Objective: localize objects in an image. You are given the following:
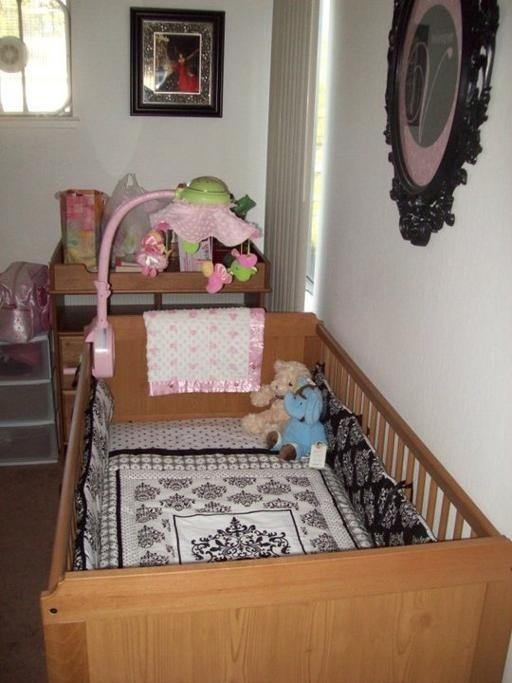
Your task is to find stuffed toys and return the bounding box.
[241,359,327,462]
[201,249,257,294]
[136,229,169,277]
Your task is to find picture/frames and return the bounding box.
[383,0,501,248]
[129,6,225,118]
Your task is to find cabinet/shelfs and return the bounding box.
[0,325,60,467]
[45,234,272,466]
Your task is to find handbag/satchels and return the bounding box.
[58,188,105,273]
[1,261,50,345]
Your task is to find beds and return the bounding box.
[37,305,512,682]
